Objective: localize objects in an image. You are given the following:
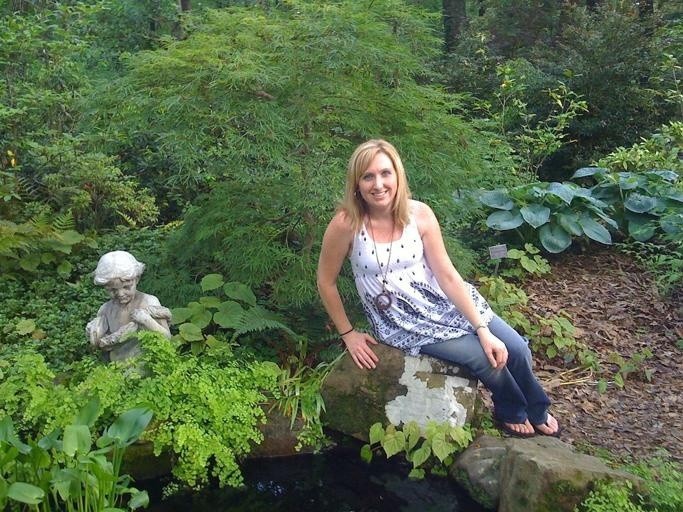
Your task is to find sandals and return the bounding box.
[501,409,536,437]
[528,410,562,437]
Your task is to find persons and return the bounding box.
[316,139,562,438]
[85,249,172,366]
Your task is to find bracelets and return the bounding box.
[339,327,354,336]
[476,323,486,332]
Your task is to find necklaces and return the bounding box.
[366,209,396,309]
